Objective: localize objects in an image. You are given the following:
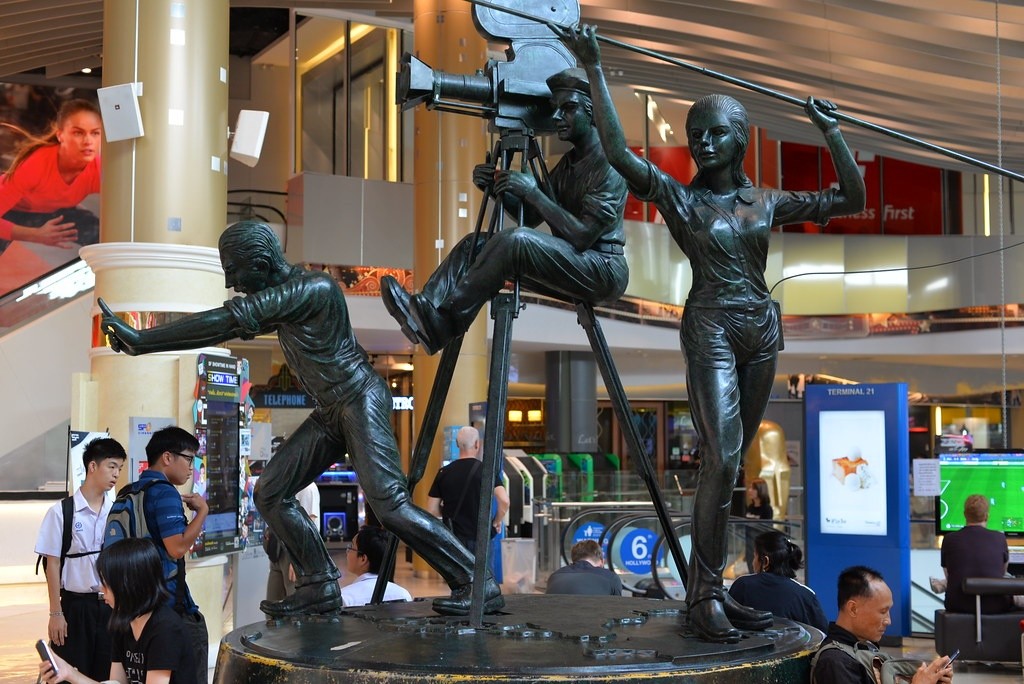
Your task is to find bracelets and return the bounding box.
[49,611,64,616]
[71,667,79,684]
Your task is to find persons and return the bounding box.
[743,477,774,573]
[729,530,832,633]
[426,427,509,578]
[927,494,1016,614]
[807,566,953,684]
[545,540,623,596]
[121,424,211,613]
[34,436,127,684]
[339,524,413,605]
[0,99,105,264]
[40,538,203,684]
[546,19,867,641]
[382,67,628,356]
[264,481,320,623]
[97,222,506,619]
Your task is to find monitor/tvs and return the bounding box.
[935,449,1024,538]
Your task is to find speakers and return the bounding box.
[229,109,271,168]
[97,84,145,143]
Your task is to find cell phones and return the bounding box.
[943,649,960,669]
[35,639,58,678]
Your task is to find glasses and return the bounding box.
[168,451,194,466]
[346,544,366,556]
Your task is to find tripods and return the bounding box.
[370,114,689,626]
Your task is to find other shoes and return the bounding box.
[929,576,946,594]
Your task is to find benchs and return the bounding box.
[935,578,1024,663]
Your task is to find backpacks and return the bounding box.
[102,479,173,552]
[810,641,925,684]
